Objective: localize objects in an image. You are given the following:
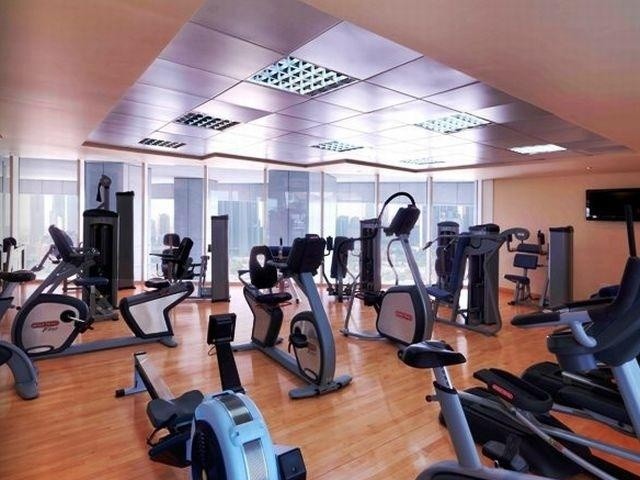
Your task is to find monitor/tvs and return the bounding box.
[586,188,640,221]
